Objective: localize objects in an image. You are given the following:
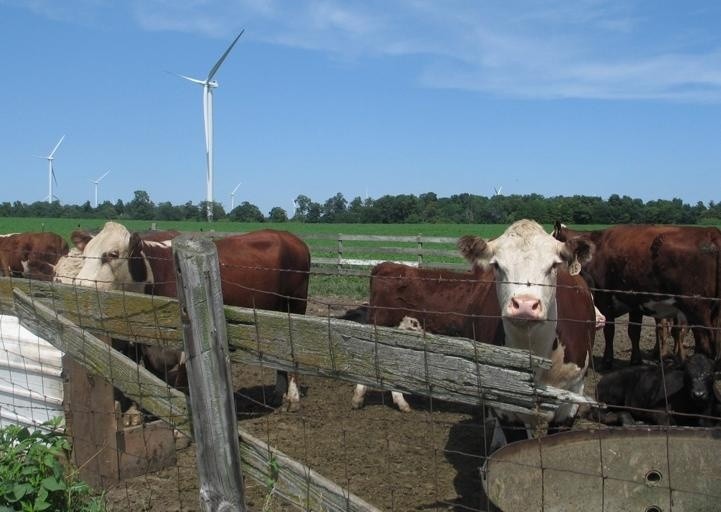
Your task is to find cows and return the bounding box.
[339,216,720,446]
[0,218,312,413]
